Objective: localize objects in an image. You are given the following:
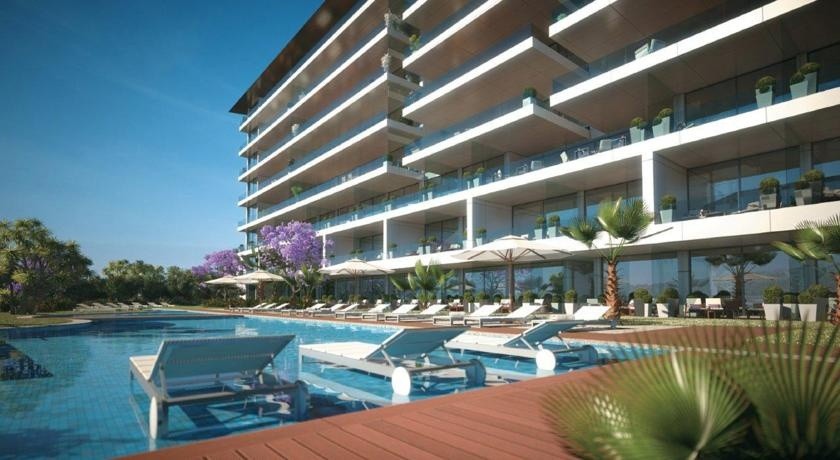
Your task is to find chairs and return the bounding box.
[76,301,176,312]
[445,320,599,370]
[229,297,764,341]
[298,326,486,396]
[129,336,308,440]
[732,201,759,214]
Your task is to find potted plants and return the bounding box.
[389,62,840,323]
